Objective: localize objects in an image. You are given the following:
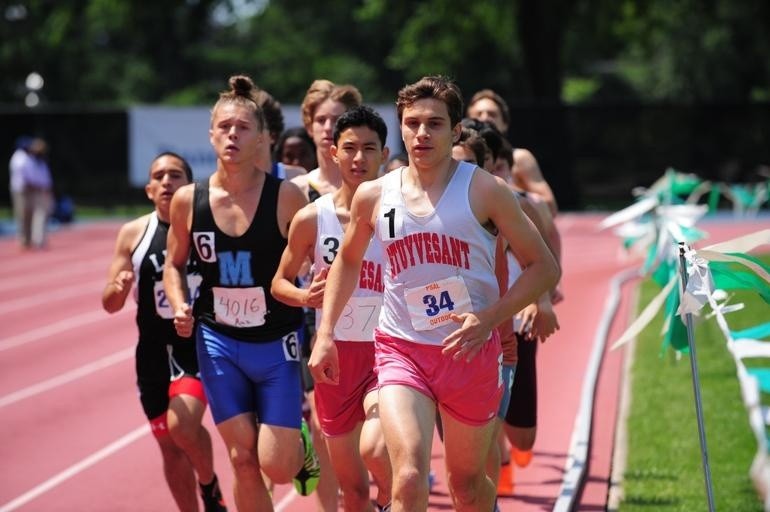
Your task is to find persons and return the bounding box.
[100,73,568,512]
[8,135,59,253]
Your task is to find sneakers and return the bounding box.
[497,463,515,496]
[511,444,533,467]
[199,474,229,512]
[294,418,321,496]
